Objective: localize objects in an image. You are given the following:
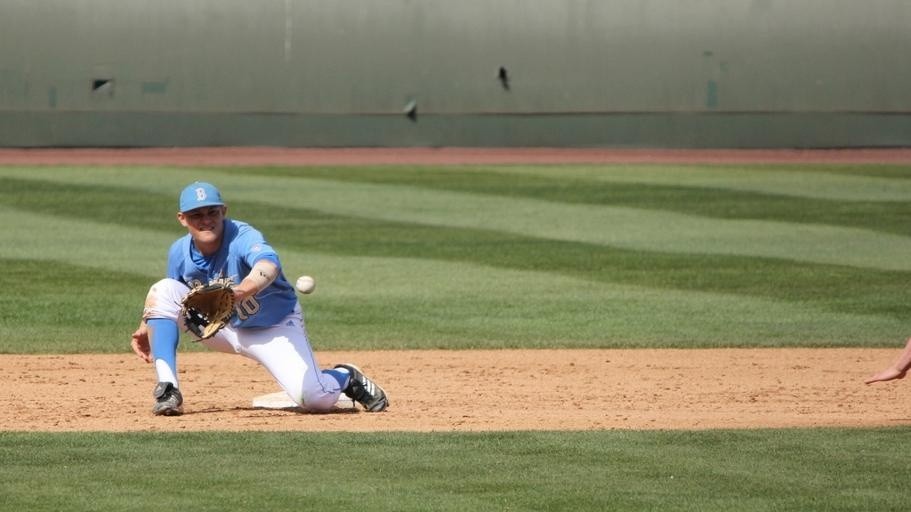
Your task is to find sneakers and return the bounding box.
[333,363,388,412]
[152,382,184,416]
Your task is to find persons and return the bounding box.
[129,179,391,416]
[863,333,911,385]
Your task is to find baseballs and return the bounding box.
[296,276,315,294]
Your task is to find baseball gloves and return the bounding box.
[174,282,234,343]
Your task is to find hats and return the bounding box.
[179,181,224,213]
